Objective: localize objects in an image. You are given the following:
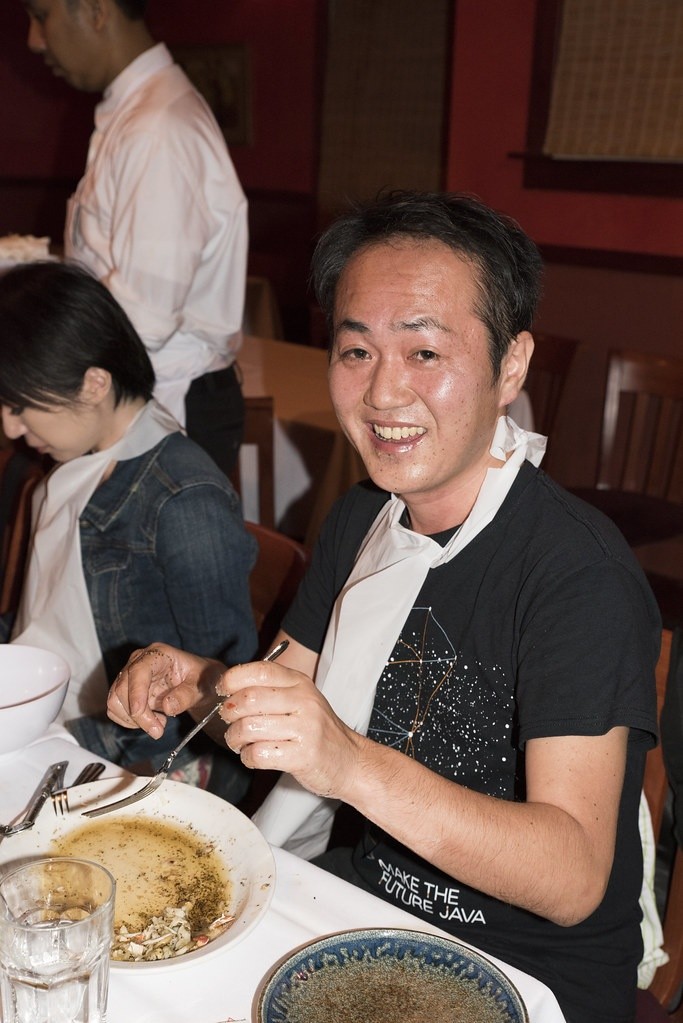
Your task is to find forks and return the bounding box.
[80,638,291,819]
[47,761,70,817]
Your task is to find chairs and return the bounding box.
[0,278,683,1023]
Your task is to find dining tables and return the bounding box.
[239,336,361,550]
[0,724,562,1023]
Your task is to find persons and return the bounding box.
[0,258,259,805]
[105,192,669,1023]
[26,0,250,479]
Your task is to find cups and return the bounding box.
[0,855,116,1023]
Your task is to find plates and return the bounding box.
[0,776,277,973]
[256,927,530,1023]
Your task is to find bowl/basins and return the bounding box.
[0,643,71,755]
